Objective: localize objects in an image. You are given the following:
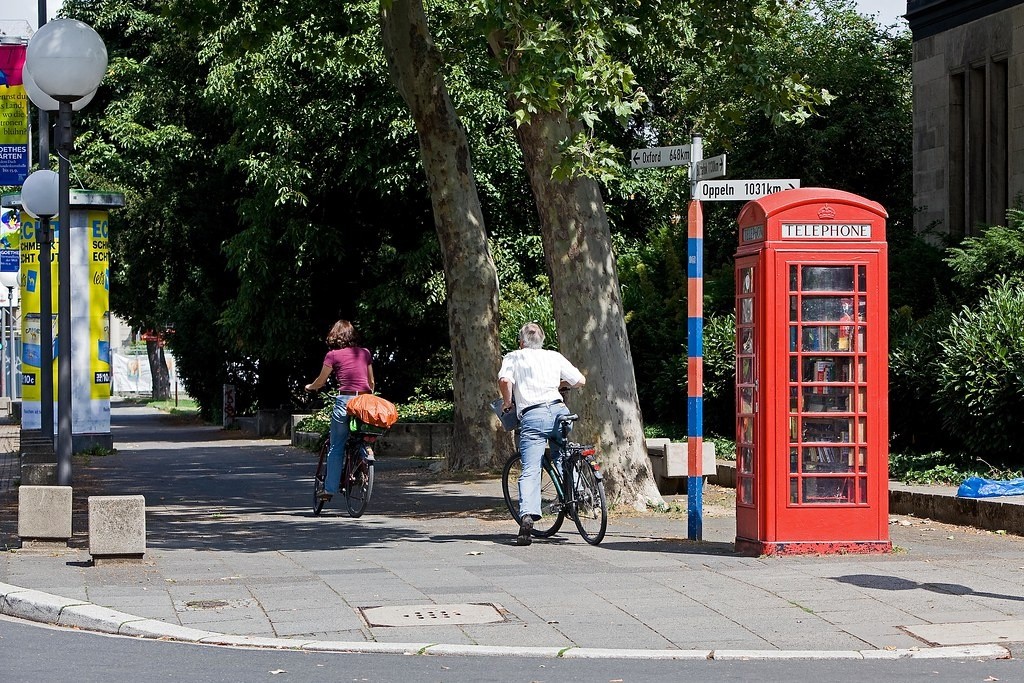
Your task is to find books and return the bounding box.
[838,297,866,351]
[813,317,839,351]
[812,359,835,394]
[807,435,836,463]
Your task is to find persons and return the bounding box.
[305,320,375,499]
[497,321,586,545]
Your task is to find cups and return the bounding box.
[812,361,831,395]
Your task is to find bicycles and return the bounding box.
[489,388,608,545]
[306,388,383,518]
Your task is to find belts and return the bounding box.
[340,390,372,395]
[521,399,563,417]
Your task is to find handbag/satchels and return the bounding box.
[346,394,399,429]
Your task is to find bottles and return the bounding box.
[837,302,851,353]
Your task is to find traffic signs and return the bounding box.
[699,179,800,201]
[631,145,690,169]
[695,154,725,179]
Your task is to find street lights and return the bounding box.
[22,19,107,484]
[21,169,59,451]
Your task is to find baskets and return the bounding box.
[491,393,519,431]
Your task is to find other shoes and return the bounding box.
[356,460,368,474]
[315,489,335,498]
[517,515,533,546]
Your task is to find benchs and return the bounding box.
[644,435,717,494]
